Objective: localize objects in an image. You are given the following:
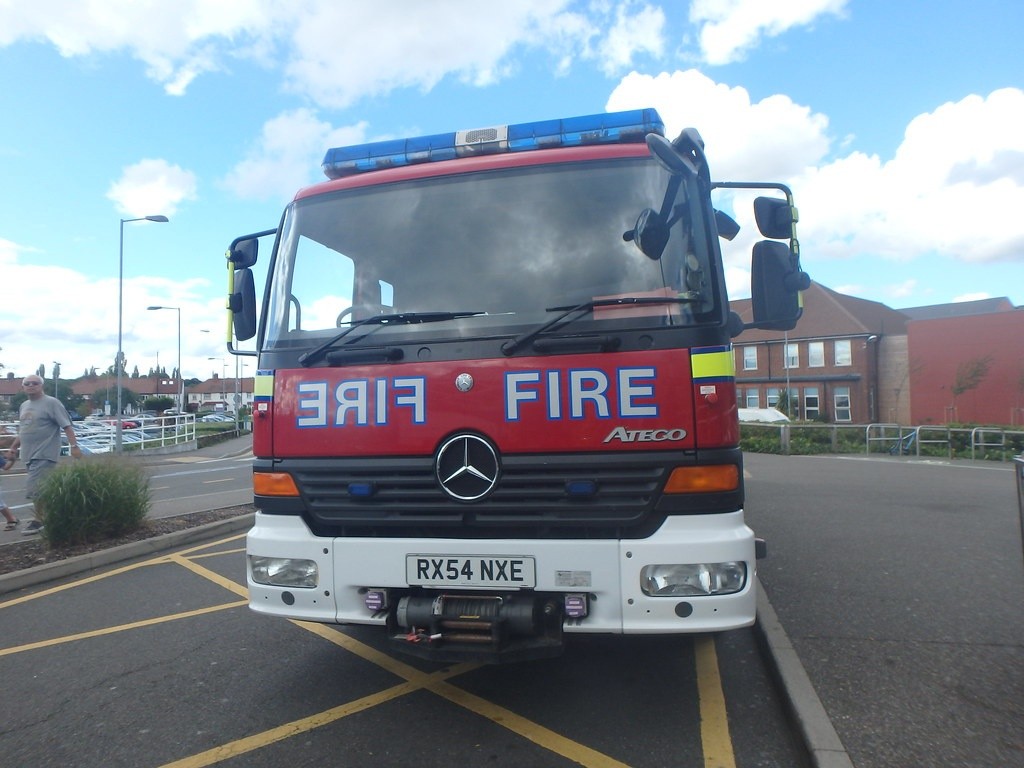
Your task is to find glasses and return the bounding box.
[23,381,43,387]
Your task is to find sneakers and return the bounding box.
[21,520,44,536]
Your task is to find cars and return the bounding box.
[0,411,237,462]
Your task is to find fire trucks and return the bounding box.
[225,107,811,669]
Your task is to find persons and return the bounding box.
[0,448,20,531]
[4,375,81,535]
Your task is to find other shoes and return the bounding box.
[5,518,19,531]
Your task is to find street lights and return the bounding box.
[53,360,61,398]
[199,328,247,435]
[147,306,181,427]
[112,215,170,455]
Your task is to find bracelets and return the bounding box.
[71,444,78,448]
